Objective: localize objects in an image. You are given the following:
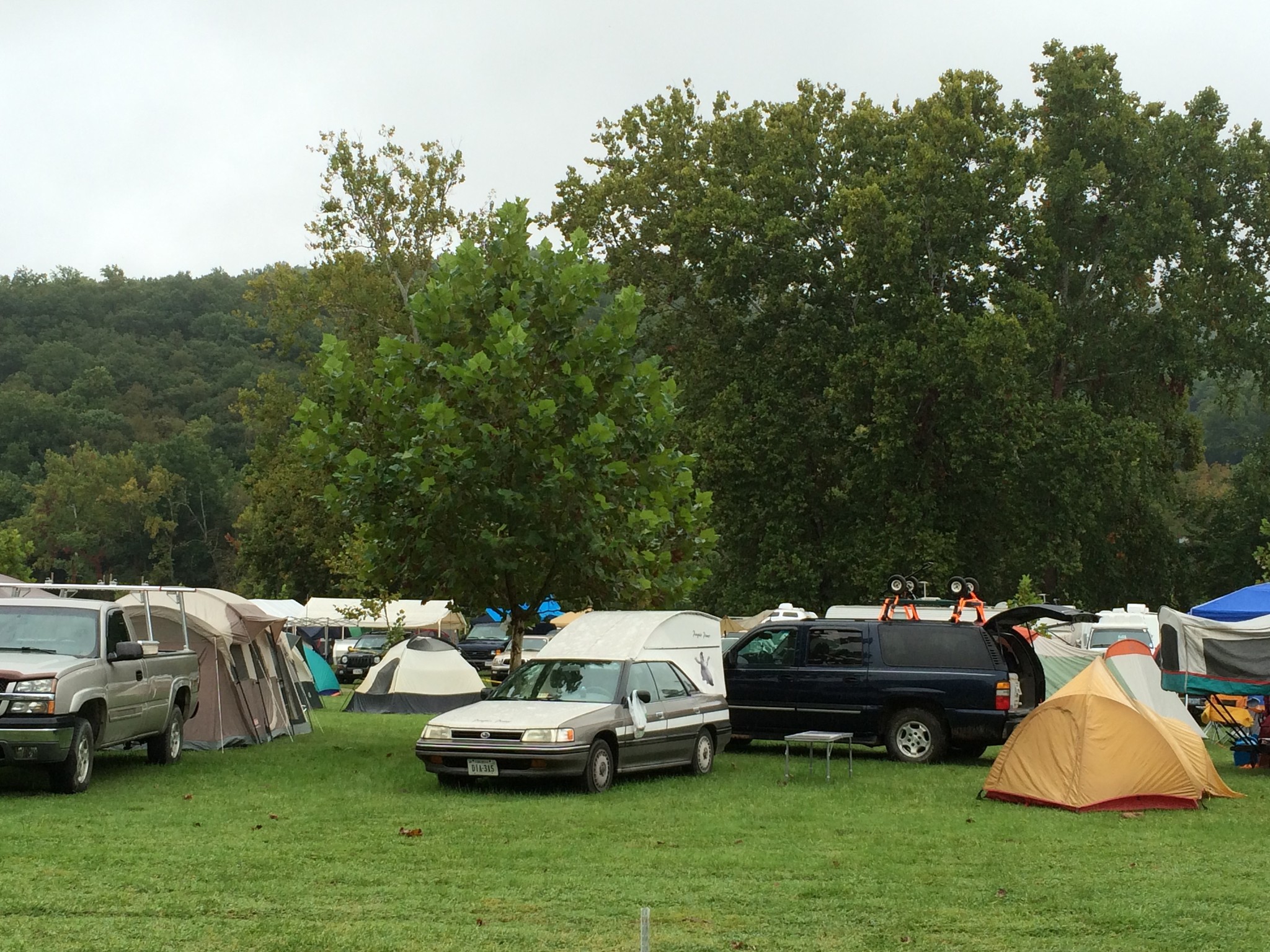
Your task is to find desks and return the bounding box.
[784,731,853,780]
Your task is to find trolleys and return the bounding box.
[878,561,987,608]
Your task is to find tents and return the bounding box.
[470,594,598,629]
[719,609,774,637]
[981,655,1250,812]
[0,573,466,754]
[343,634,490,713]
[1007,578,1270,750]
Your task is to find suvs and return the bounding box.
[334,630,414,684]
[720,604,1102,765]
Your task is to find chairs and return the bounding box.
[1203,696,1264,746]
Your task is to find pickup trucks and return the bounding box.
[0,579,203,796]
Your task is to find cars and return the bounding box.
[413,654,733,795]
[1078,624,1156,657]
[456,621,513,669]
[488,635,553,687]
